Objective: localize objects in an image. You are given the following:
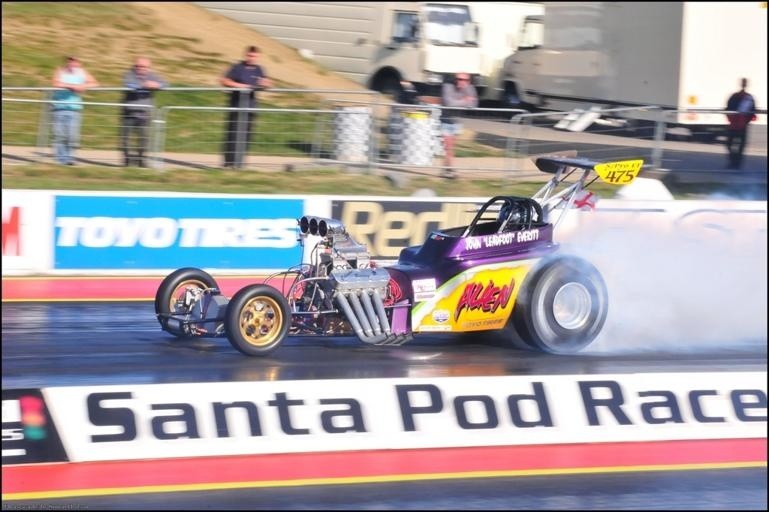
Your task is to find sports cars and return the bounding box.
[154,153,645,358]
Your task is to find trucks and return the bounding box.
[502,2,768,137]
[188,3,487,103]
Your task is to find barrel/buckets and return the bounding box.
[330,104,375,173]
[386,106,444,169]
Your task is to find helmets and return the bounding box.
[497,198,543,232]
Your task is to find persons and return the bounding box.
[219,43,274,169]
[434,70,481,180]
[47,53,100,169]
[117,57,170,170]
[721,77,757,171]
[492,204,532,233]
